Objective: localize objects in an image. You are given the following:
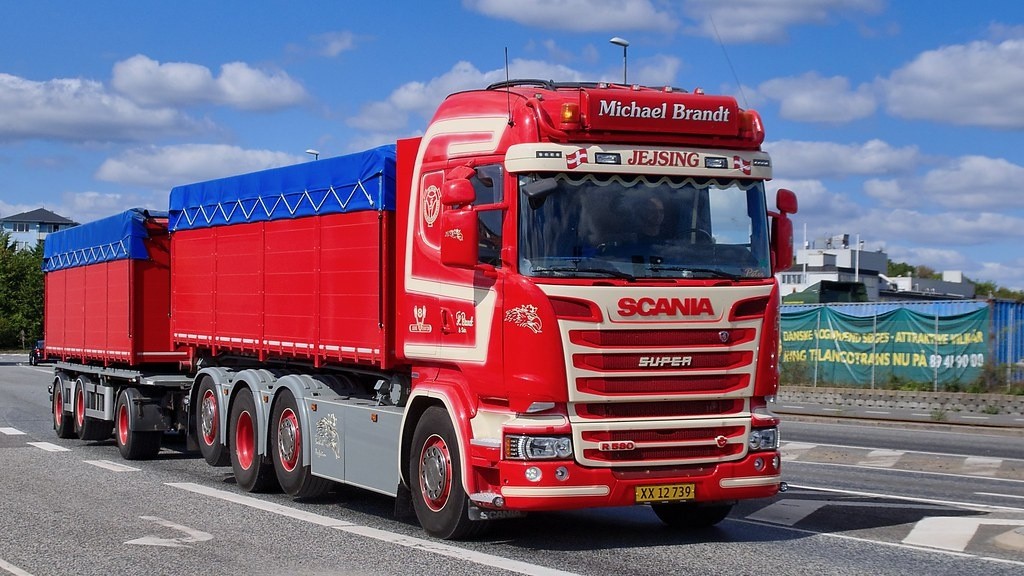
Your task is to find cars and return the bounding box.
[29,339,62,366]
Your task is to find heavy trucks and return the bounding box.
[37,80,801,543]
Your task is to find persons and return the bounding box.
[640,197,665,246]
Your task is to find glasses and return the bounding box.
[647,207,666,214]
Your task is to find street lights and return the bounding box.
[609,36,630,85]
